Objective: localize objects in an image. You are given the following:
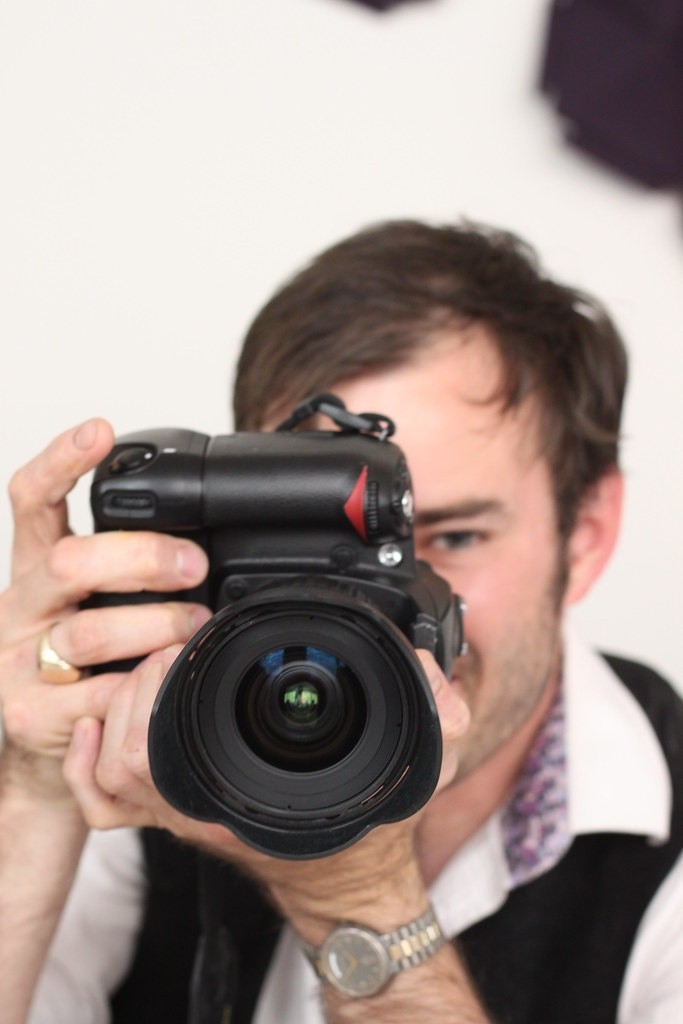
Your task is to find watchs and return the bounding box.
[303,904,446,1003]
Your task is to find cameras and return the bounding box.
[76,424,464,860]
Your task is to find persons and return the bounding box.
[0,209,683,1024]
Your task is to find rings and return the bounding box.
[37,619,81,687]
